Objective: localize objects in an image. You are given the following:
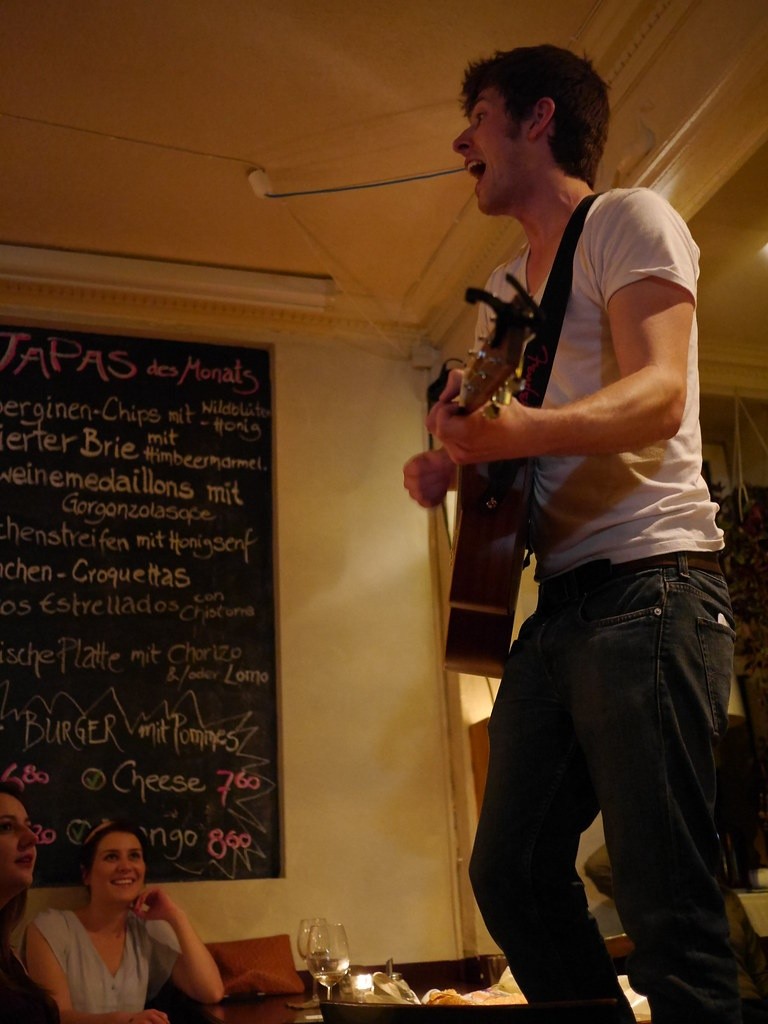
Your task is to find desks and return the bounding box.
[195,974,489,1024]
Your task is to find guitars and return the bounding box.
[441,272,543,679]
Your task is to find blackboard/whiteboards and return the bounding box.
[0,313,289,884]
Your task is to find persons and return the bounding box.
[1,780,223,1024]
[403,45,768,1024]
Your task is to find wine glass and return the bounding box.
[307,923,350,1001]
[298,917,330,1003]
